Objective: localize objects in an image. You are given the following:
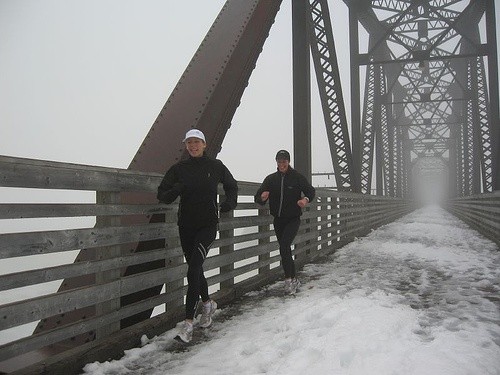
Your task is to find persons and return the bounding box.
[156,129,239,343]
[254,150,316,297]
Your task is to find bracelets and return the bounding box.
[304,197,309,203]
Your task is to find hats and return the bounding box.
[276,150,290,161]
[182,129,206,143]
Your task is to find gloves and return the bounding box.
[173,182,184,193]
[219,201,235,213]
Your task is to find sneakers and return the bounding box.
[284,277,300,295]
[199,299,217,327]
[173,322,193,342]
